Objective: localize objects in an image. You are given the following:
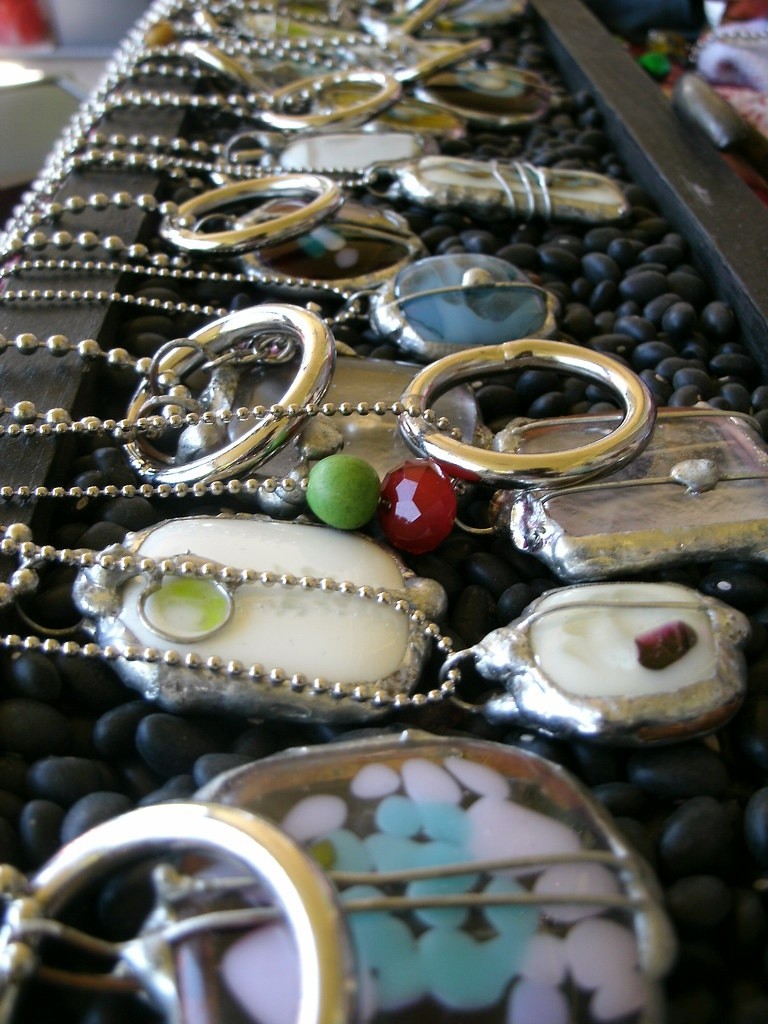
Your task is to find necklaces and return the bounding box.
[0,1,767,1024]
